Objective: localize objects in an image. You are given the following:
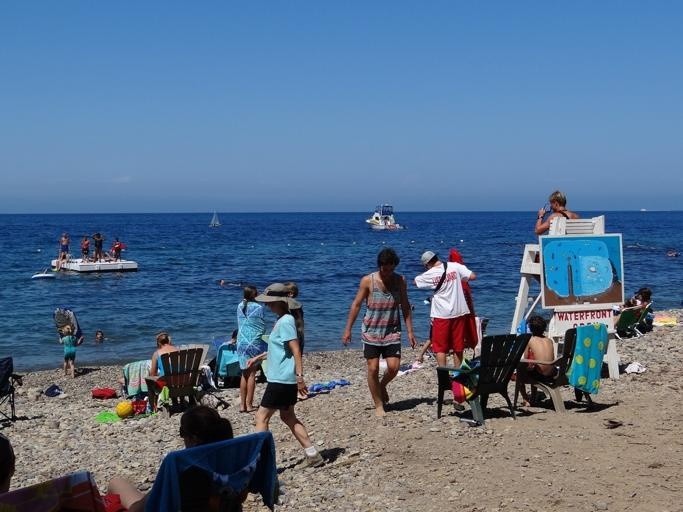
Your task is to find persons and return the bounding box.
[236,287,260,413]
[58,325,81,374]
[412,250,475,370]
[342,249,417,418]
[418,316,432,365]
[95,330,105,343]
[613,286,653,330]
[216,329,237,361]
[534,190,577,262]
[149,331,187,411]
[59,233,125,262]
[247,284,322,468]
[516,315,556,406]
[282,284,306,400]
[0,433,14,493]
[107,405,232,511]
[219,279,225,286]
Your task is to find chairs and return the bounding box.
[145,348,204,418]
[520,213,606,275]
[618,301,654,338]
[145,432,280,511]
[513,324,607,413]
[436,334,532,424]
[0,356,24,423]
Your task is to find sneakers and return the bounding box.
[294,451,323,470]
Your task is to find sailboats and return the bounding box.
[209,209,223,229]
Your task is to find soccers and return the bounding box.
[116,401,134,418]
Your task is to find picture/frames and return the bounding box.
[539,232,624,310]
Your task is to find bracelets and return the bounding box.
[537,216,543,219]
[295,373,304,377]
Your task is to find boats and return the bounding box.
[365,203,405,232]
[51,256,138,273]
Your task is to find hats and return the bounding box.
[421,251,438,266]
[635,288,651,294]
[255,283,302,310]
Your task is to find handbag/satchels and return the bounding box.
[92,388,116,400]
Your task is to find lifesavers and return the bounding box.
[32,273,56,279]
